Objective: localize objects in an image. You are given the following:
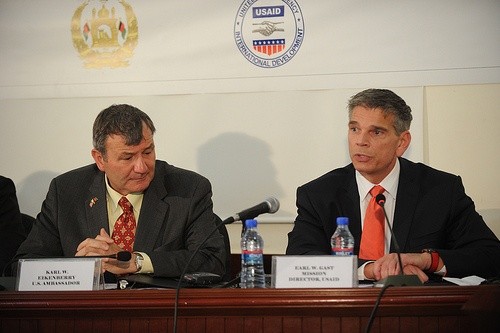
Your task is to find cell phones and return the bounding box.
[184,273,222,284]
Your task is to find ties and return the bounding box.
[110,197,136,253]
[359,184,386,261]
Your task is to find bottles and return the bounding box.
[240,220,265,289]
[330,217,355,255]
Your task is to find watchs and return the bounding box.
[135,253,145,271]
[421,248,439,272]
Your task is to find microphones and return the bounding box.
[374,194,424,287]
[224,197,280,224]
[0,250,132,291]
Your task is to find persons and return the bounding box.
[285,88,500,283]
[0,175,28,277]
[7,104,231,286]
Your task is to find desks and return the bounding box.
[0,279,500,333]
